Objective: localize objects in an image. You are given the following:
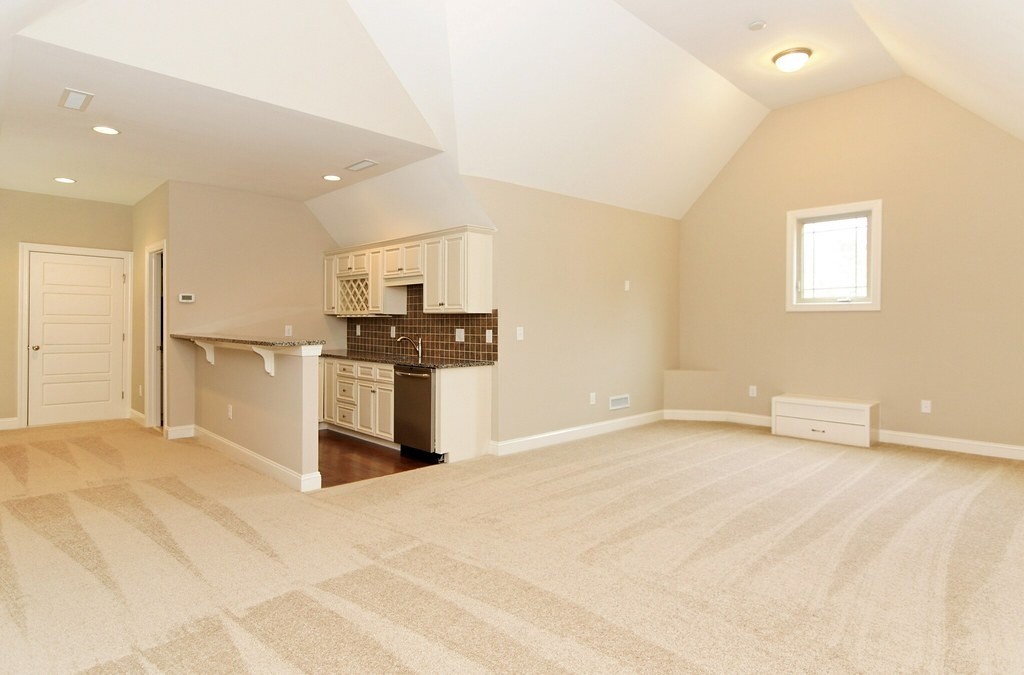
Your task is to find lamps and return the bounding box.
[772,47,813,72]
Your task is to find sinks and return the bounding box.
[380,355,426,364]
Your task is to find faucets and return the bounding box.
[397,336,421,363]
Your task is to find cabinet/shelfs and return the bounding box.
[771,393,880,449]
[319,348,495,463]
[322,224,498,316]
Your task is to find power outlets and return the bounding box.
[356,325,360,335]
[749,385,757,397]
[485,329,493,343]
[590,392,596,404]
[285,325,292,336]
[517,327,524,341]
[391,326,396,338]
[921,400,932,413]
[455,329,464,341]
